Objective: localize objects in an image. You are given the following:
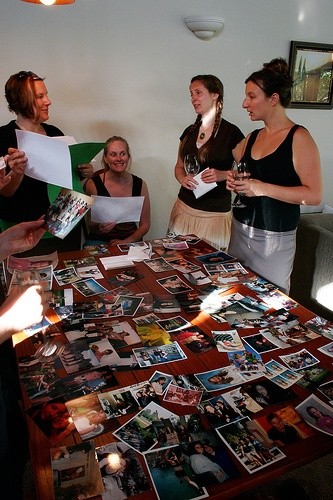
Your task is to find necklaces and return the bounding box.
[199,121,215,139]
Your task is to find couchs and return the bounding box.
[287,212,332,323]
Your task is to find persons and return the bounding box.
[165,74,247,252]
[0,70,94,258]
[0,215,51,345]
[7,186,333,500]
[83,136,150,246]
[225,57,322,298]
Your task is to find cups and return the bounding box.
[183,153,200,179]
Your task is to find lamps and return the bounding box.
[184,15,225,41]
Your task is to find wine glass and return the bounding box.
[231,160,249,207]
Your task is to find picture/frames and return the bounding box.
[284,40,333,109]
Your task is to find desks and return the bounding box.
[6,232,333,500]
